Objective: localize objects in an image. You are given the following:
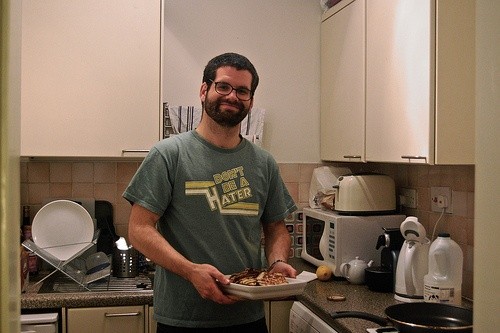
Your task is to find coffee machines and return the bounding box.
[367,226,405,291]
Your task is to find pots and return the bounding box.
[330,302,473,333]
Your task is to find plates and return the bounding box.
[216,275,306,299]
[32,200,94,261]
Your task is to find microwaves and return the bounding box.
[301,208,406,276]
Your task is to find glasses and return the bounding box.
[209,79,254,101]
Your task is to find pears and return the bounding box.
[316,265,331,281]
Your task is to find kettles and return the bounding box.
[394,216,431,303]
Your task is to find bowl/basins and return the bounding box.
[86,252,110,274]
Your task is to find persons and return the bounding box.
[122,53,297,332]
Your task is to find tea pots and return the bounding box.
[340,256,374,284]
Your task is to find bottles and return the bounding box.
[22,206,37,281]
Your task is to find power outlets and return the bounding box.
[430,186,453,214]
[399,189,417,209]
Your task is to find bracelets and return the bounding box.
[268,260,286,271]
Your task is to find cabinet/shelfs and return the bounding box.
[319,0,476,165]
[21,0,164,162]
[21,258,474,333]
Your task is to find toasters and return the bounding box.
[333,173,396,215]
